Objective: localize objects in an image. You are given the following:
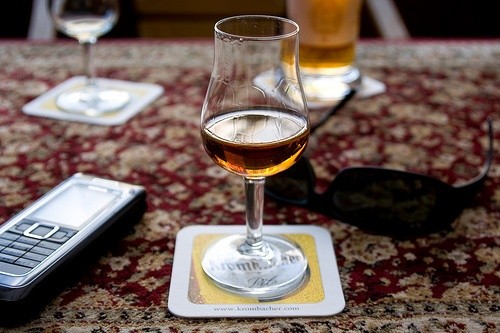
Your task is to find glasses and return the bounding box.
[264,87,493,240]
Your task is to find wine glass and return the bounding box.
[200,16,309,299]
[52,0,132,115]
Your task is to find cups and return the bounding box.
[278,0,361,87]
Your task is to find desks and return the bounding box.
[0,38,500,333]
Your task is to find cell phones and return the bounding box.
[0,172,147,302]
